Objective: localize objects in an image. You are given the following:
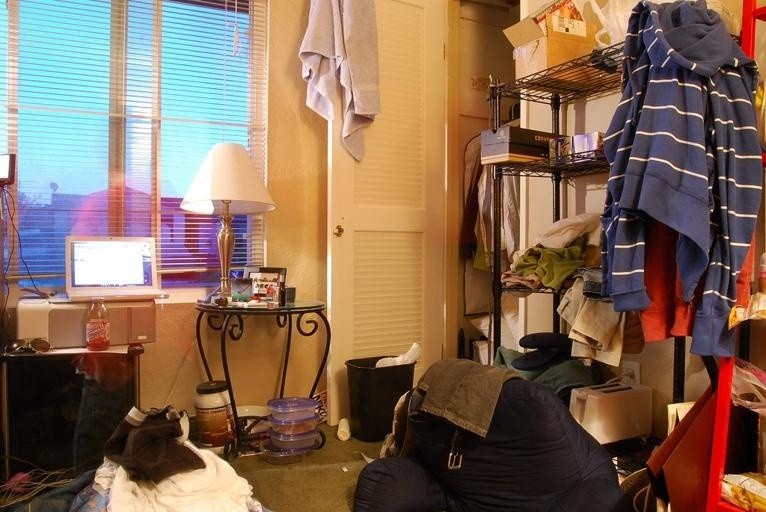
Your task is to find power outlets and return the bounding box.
[621,361,641,385]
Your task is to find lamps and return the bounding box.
[178,142,277,298]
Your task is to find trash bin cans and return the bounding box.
[344,355,417,442]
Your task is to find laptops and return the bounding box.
[64,234,170,301]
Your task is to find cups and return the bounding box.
[285,285,296,304]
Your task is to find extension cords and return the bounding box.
[47,293,68,303]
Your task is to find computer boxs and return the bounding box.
[15,298,157,350]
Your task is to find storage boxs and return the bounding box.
[502,11,598,96]
[479,126,606,165]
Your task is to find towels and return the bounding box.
[298,0,380,164]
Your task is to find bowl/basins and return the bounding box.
[230,405,272,436]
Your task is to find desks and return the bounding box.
[1,345,145,480]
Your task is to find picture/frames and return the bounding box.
[242,266,287,302]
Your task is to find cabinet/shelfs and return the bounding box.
[487,38,719,402]
[707,356,766,512]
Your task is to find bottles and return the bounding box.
[84,295,112,351]
[192,381,240,456]
[277,272,287,307]
